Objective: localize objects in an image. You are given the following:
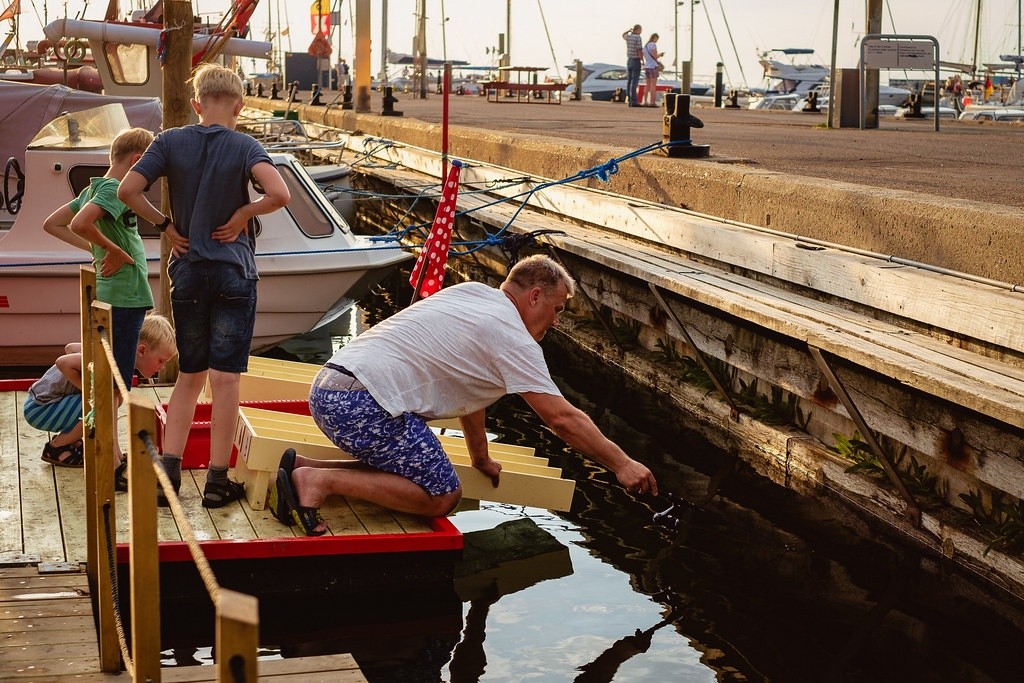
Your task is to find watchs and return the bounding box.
[153,215,171,232]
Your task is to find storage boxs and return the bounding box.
[638,84,673,104]
[154,399,309,469]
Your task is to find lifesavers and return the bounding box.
[64,39,87,62]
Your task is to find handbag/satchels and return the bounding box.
[656,61,665,72]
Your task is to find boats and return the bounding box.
[0,0,415,378]
[566,45,1023,123]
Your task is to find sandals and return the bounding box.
[114,462,128,494]
[157,479,181,507]
[202,477,245,508]
[52,434,83,455]
[41,441,85,468]
[120,452,128,464]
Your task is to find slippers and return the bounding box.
[277,466,327,536]
[268,448,296,524]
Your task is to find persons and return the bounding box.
[116,64,290,507]
[644,33,663,107]
[339,59,348,91]
[622,24,644,107]
[24,315,178,467]
[269,253,657,537]
[43,128,156,490]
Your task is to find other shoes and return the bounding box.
[642,103,648,106]
[629,103,643,107]
[648,103,659,108]
